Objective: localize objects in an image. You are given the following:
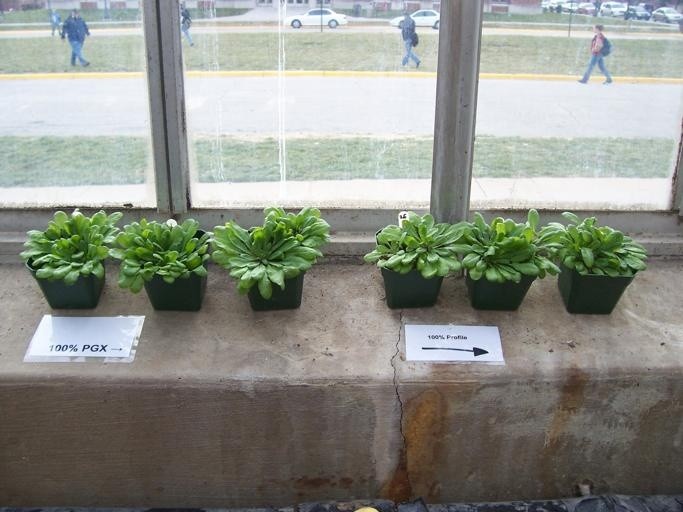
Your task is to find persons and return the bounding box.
[578,24,613,83]
[61,10,90,68]
[180,3,196,46]
[49,9,61,37]
[399,10,421,68]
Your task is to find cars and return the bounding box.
[651,7,682,22]
[540,1,653,21]
[389,9,440,30]
[283,8,347,28]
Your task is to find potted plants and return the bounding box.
[20,206,121,308]
[550,210,648,317]
[461,210,560,310]
[205,204,334,313]
[364,209,473,309]
[111,215,212,309]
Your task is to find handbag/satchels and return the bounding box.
[410,32,419,47]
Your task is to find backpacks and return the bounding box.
[601,36,613,57]
